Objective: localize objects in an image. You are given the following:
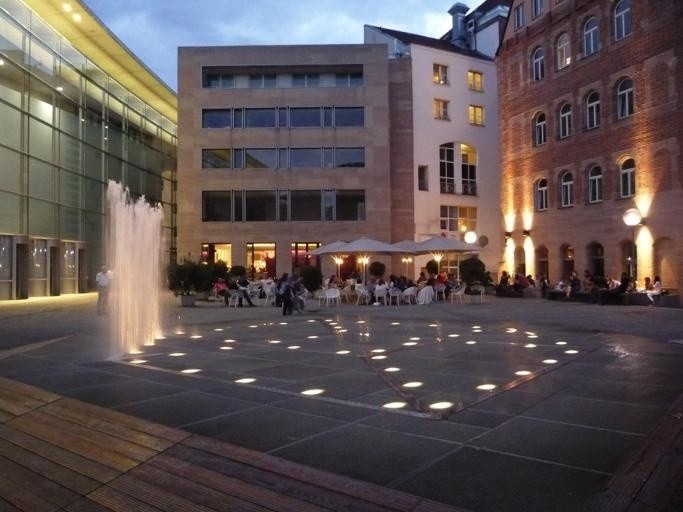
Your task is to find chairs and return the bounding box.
[211,275,486,310]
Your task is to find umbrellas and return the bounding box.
[303,237,486,289]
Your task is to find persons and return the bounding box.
[95,266,112,316]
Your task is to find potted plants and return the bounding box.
[163,250,227,307]
